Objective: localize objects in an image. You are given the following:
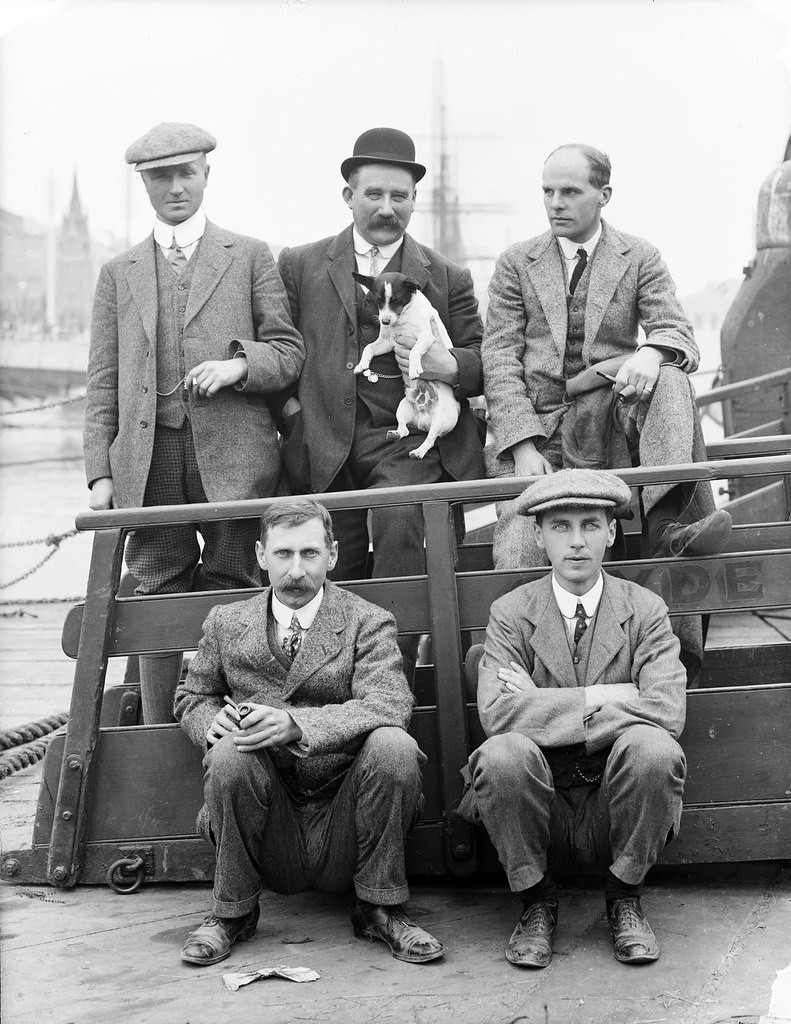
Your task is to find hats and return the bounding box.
[126,122,216,171]
[341,128,426,182]
[514,467,633,517]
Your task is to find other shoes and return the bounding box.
[464,644,484,703]
[649,509,732,560]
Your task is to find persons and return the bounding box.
[466,141,732,702]
[278,128,484,703]
[181,498,445,965]
[456,467,687,969]
[83,122,307,724]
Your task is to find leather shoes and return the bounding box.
[350,900,446,963]
[505,900,556,966]
[607,896,660,961]
[180,902,261,965]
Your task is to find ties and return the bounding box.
[281,616,302,660]
[574,603,588,645]
[167,237,187,273]
[369,248,379,277]
[569,249,587,296]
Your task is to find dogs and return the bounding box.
[352,268,462,459]
